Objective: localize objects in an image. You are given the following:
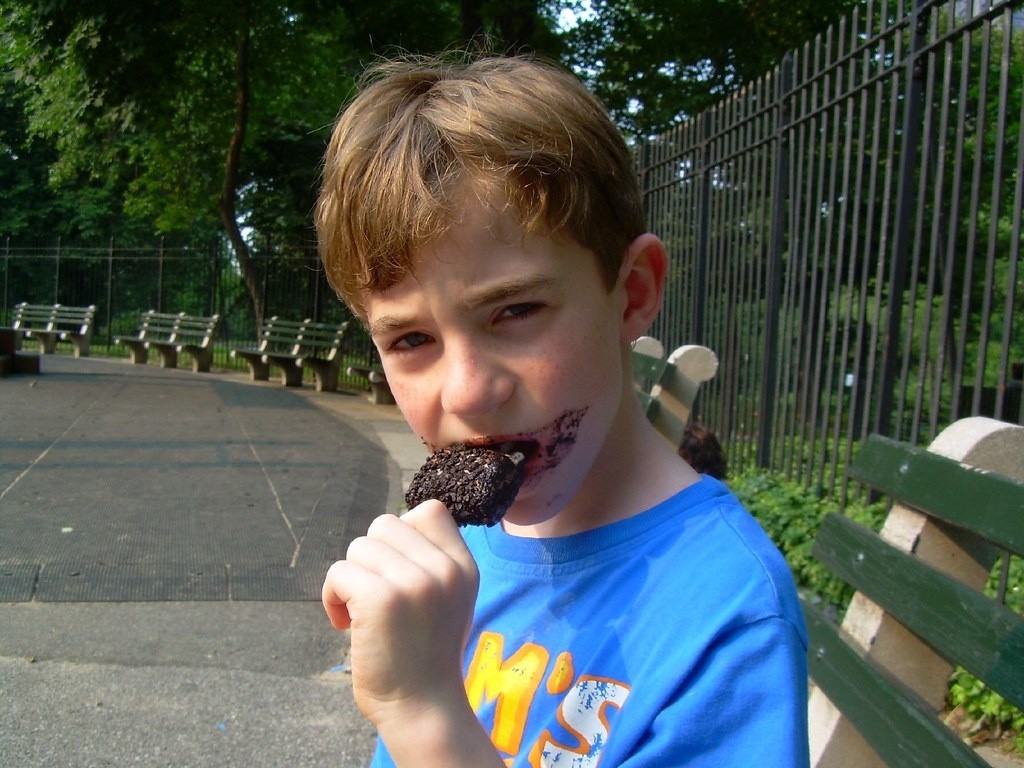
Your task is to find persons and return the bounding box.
[318,48,811,768]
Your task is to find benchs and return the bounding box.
[113,309,222,373]
[0,302,96,358]
[794,414,1024,768]
[231,316,352,391]
[631,335,718,452]
[345,338,395,404]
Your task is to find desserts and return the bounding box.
[400,440,529,521]
